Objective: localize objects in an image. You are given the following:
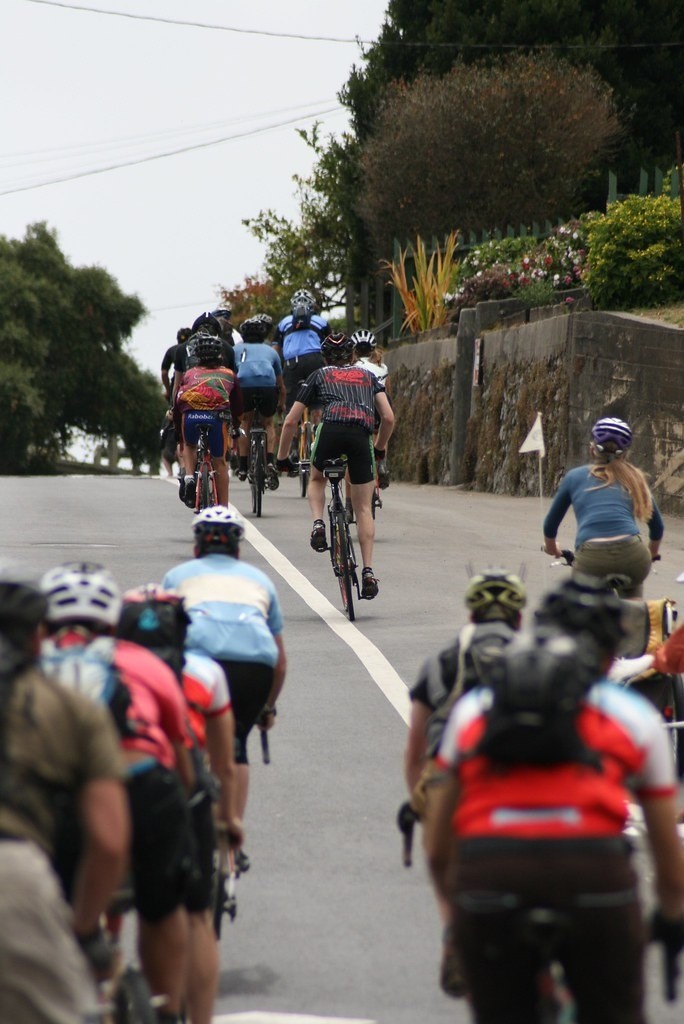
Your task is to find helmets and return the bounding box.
[193,505,243,541]
[0,560,47,623]
[253,313,271,326]
[176,327,193,344]
[193,313,221,331]
[238,318,267,335]
[319,334,354,360]
[350,328,377,350]
[195,336,222,357]
[116,584,186,636]
[212,308,231,321]
[42,561,120,624]
[290,290,316,313]
[593,416,633,447]
[465,571,525,616]
[539,584,622,658]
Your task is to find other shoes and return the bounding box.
[310,525,326,550]
[362,574,378,596]
[232,850,250,873]
[178,476,186,499]
[185,479,196,507]
[377,460,390,490]
[266,463,280,489]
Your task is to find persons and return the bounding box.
[272,288,333,476]
[160,504,286,875]
[403,567,684,1023]
[162,301,286,508]
[0,563,245,1024]
[351,328,391,489]
[542,417,663,599]
[276,331,395,596]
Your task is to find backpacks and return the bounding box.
[452,634,605,777]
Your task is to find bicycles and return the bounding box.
[213,706,268,937]
[246,396,274,517]
[194,421,218,514]
[96,883,166,1024]
[396,793,424,870]
[289,401,384,622]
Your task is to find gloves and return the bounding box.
[277,459,293,472]
